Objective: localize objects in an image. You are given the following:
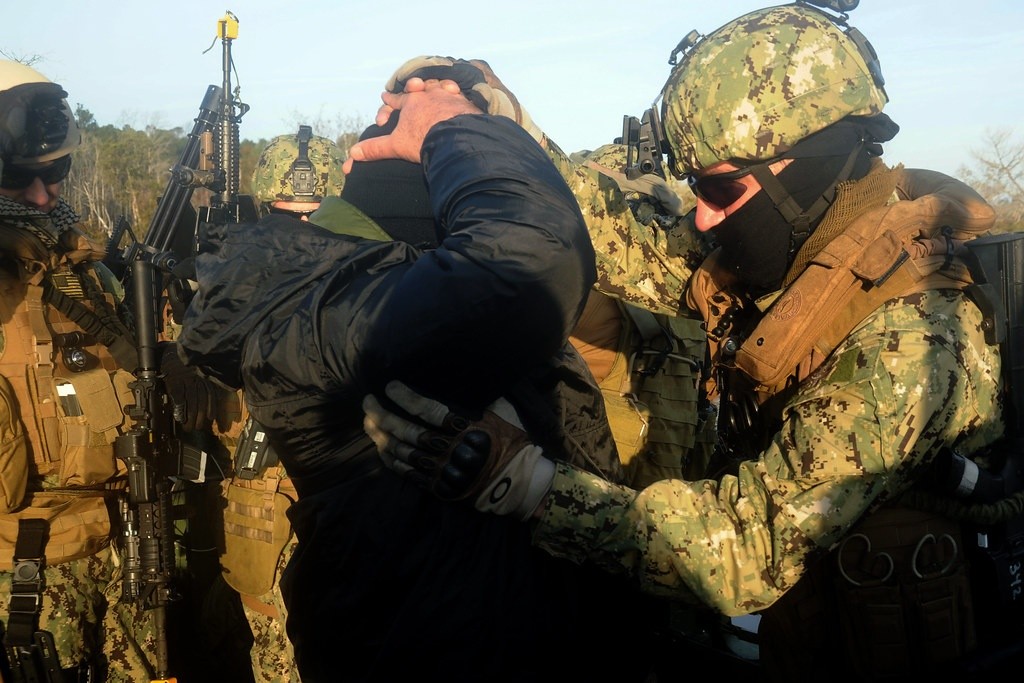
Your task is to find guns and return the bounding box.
[114,260,208,683]
[101,8,249,312]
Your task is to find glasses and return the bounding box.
[687,153,787,210]
[0,153,75,190]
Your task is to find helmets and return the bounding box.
[249,125,347,202]
[581,144,670,200]
[0,60,82,163]
[622,0,889,180]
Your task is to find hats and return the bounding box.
[340,110,447,248]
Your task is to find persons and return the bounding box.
[159,125,348,683]
[361,0,1024,683]
[0,81,217,683]
[177,53,672,683]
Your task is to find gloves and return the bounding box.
[0,82,69,157]
[362,380,543,516]
[386,55,545,147]
[156,339,218,430]
